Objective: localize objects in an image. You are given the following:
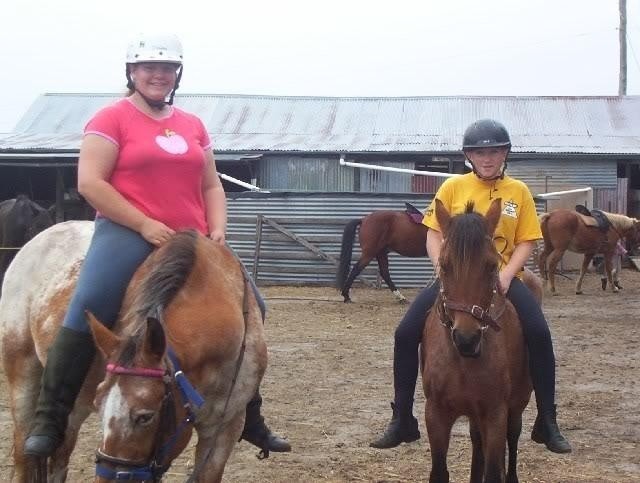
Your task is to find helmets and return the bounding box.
[125,29,184,66]
[462,119,512,151]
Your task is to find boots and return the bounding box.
[531,402,572,453]
[241,400,291,452]
[370,397,420,448]
[22,319,117,459]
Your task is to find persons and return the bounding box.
[23,38,294,459]
[367,117,573,454]
[600,241,629,290]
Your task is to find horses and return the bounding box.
[420,198,543,483]
[0,195,61,300]
[537,209,640,297]
[0,219,268,483]
[335,209,429,305]
